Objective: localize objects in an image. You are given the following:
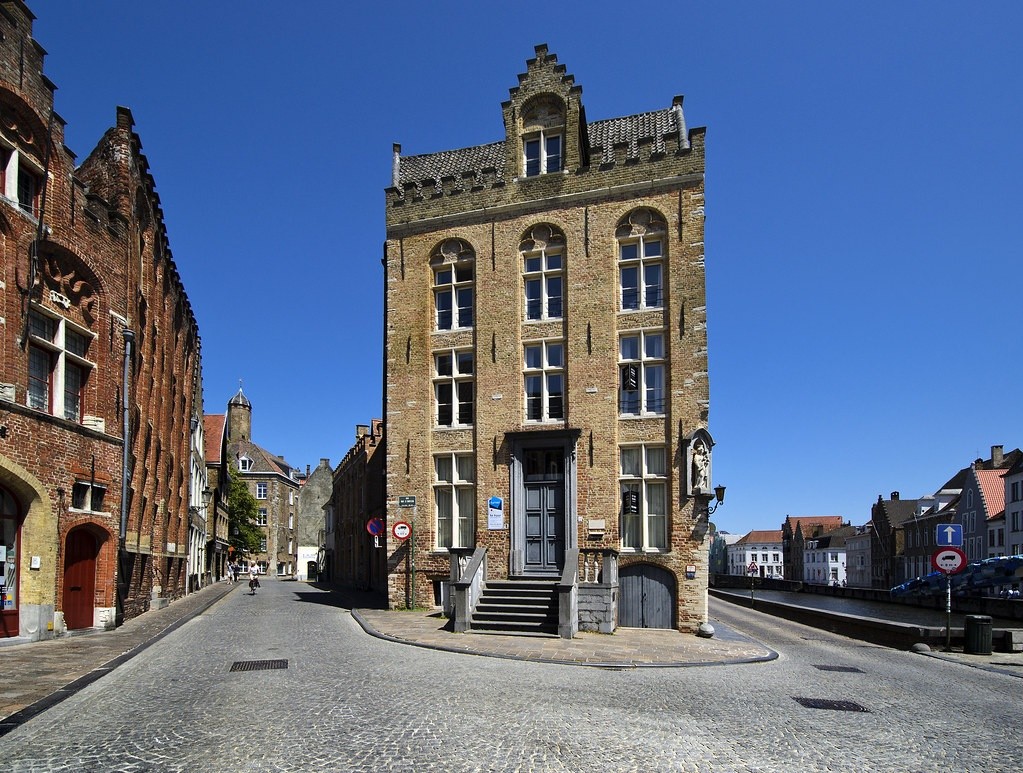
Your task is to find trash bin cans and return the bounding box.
[963,614,993,656]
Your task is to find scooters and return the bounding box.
[249,573,260,595]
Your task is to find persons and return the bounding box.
[250,561,260,591]
[226,564,240,584]
[1002,587,1020,599]
[694,445,706,489]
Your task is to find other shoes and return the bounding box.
[230,583,232,585]
[227,582,229,584]
